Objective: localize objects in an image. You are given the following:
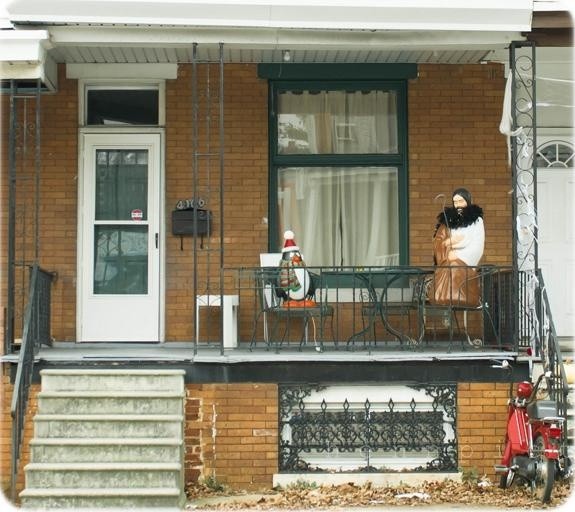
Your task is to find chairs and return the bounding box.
[247,250,502,352]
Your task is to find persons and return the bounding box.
[430,188,486,307]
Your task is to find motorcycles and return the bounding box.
[488,355,571,503]
